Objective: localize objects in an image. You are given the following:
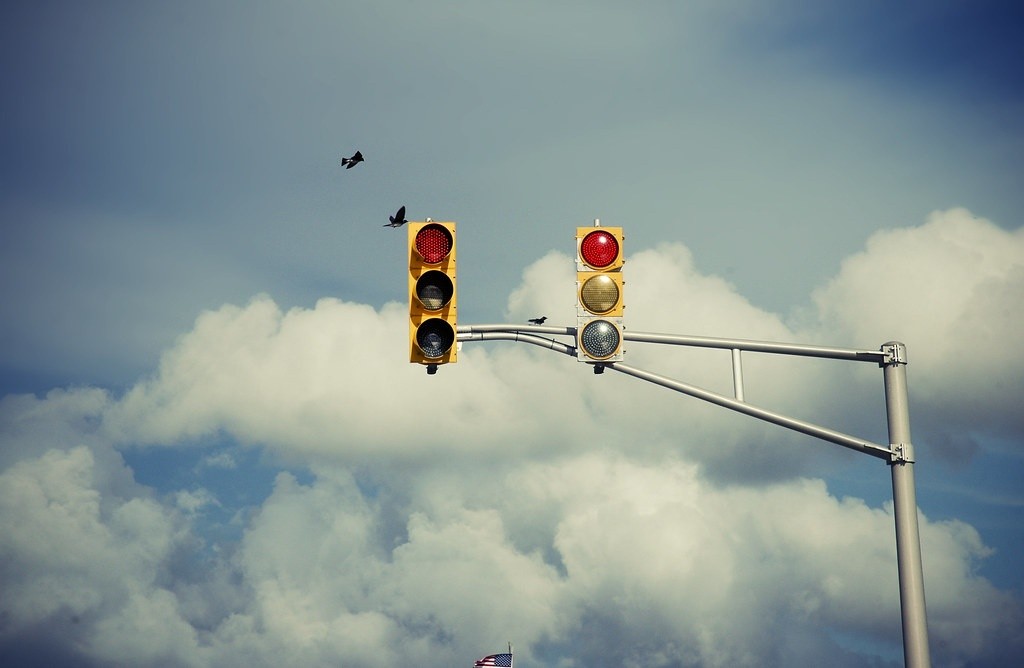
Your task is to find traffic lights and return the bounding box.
[407,221,457,364]
[575,227,625,363]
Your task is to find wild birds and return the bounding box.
[527,315,549,326]
[381,204,410,231]
[340,149,366,171]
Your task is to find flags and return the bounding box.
[473,654,511,667]
